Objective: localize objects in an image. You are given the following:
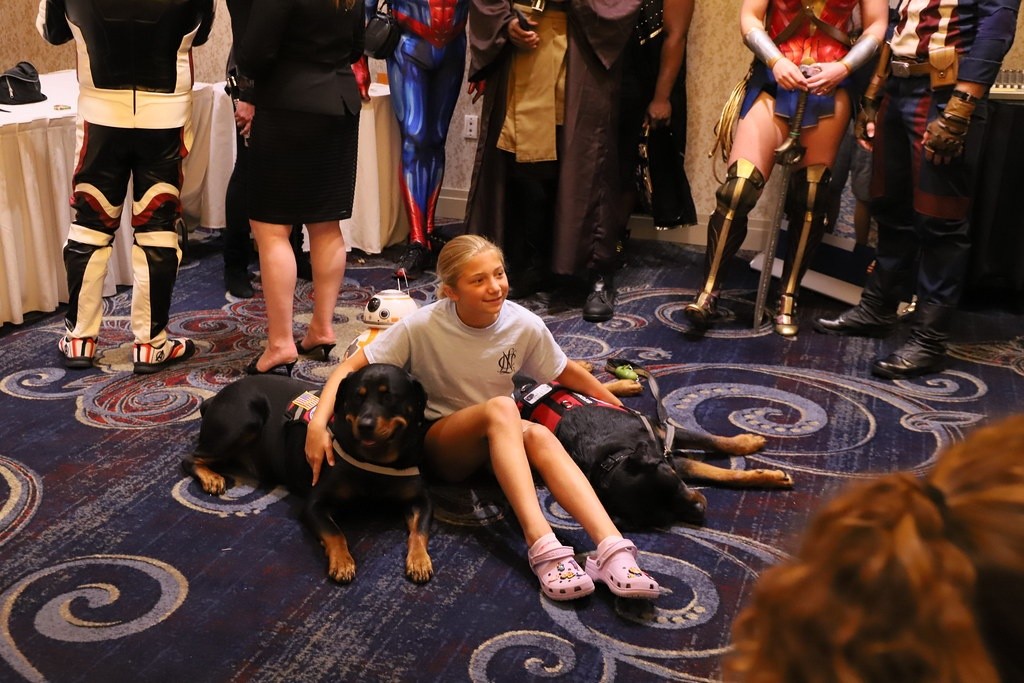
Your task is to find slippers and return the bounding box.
[530,546,595,600]
[586,538,660,599]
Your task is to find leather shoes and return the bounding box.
[583,273,614,321]
[535,275,572,300]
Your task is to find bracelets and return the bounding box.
[53,104,72,110]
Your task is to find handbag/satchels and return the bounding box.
[364,0,404,61]
[0,61,47,105]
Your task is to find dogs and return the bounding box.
[182,362,433,582]
[510,360,795,520]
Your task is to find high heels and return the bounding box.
[294,340,337,361]
[243,351,298,377]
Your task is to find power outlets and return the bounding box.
[461,114,479,138]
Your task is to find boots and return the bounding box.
[771,164,832,335]
[871,295,952,380]
[810,272,902,339]
[683,158,765,330]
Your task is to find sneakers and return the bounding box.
[392,241,423,279]
[132,337,196,374]
[57,335,98,368]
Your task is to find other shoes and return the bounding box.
[294,253,313,282]
[224,275,254,299]
[853,243,876,255]
[507,271,535,298]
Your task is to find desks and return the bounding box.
[0,65,212,330]
[204,77,395,251]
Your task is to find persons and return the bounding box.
[463,0,699,322]
[683,0,1024,381]
[36,0,217,375]
[224,1,366,379]
[352,0,485,280]
[722,410,1024,683]
[304,235,661,601]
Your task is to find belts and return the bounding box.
[890,55,968,78]
